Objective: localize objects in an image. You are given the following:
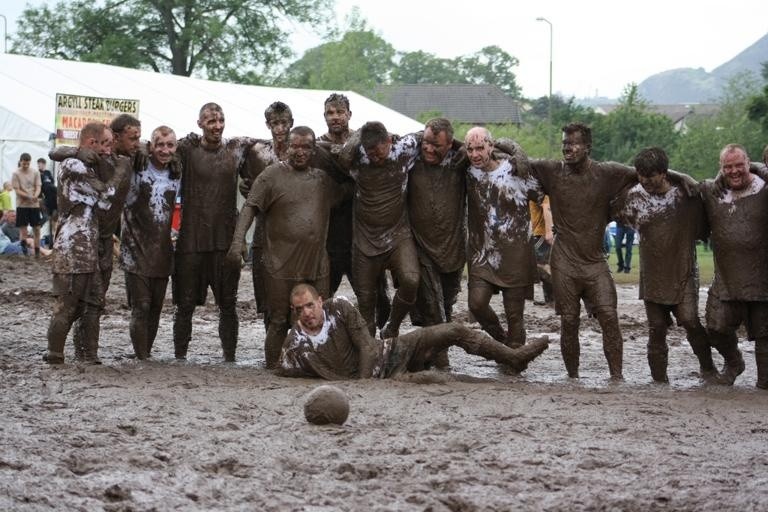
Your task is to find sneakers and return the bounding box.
[719,358,745,386]
[512,335,548,373]
[617,261,630,273]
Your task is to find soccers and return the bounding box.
[304,385,349,425]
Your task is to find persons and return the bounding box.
[0,90,768,393]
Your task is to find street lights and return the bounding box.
[536,15,553,159]
